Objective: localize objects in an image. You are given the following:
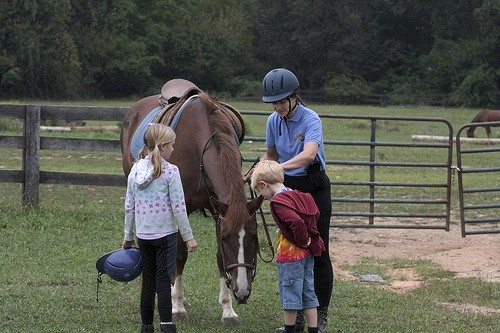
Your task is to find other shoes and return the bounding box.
[308,326,318,333]
[275,309,306,333]
[140,324,154,333]
[317,306,328,333]
[160,324,178,333]
[284,325,296,333]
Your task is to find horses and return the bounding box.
[119,93,265,327]
[467,109,500,138]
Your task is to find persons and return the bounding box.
[122,124,198,333]
[251,160,326,333]
[242,69,333,333]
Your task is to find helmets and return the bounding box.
[262,68,300,103]
[96,247,143,282]
